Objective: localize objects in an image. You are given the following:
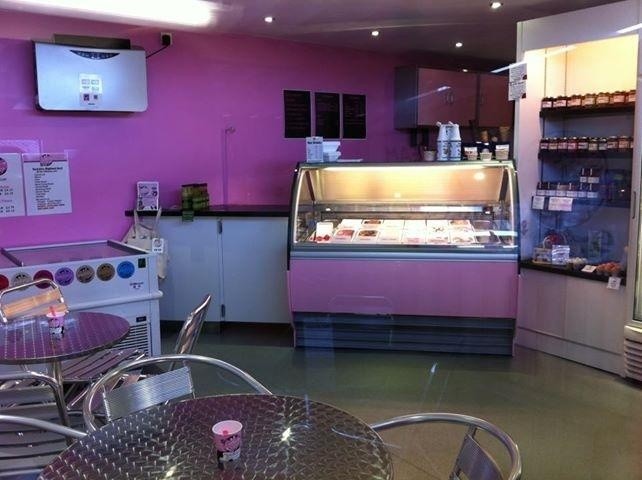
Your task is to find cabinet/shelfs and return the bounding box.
[140,216,219,322]
[479,72,514,128]
[220,218,290,325]
[514,0,642,380]
[288,157,520,360]
[393,64,479,129]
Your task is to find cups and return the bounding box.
[213,420,243,469]
[46,312,65,335]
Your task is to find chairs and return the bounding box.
[0,280,144,416]
[0,415,86,480]
[372,411,521,480]
[82,354,269,432]
[1,372,95,474]
[67,295,212,414]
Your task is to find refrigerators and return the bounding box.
[0,238,164,358]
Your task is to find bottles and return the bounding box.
[607,136,619,148]
[609,93,614,104]
[549,137,557,149]
[588,184,599,201]
[556,182,567,196]
[599,138,607,149]
[579,168,588,183]
[629,90,636,103]
[589,136,598,150]
[482,143,492,153]
[437,125,449,161]
[566,181,578,197]
[624,93,629,103]
[449,124,461,161]
[578,137,588,149]
[614,92,621,105]
[540,138,549,149]
[578,183,587,199]
[619,136,629,148]
[547,97,553,108]
[630,136,633,148]
[541,97,547,110]
[599,92,603,105]
[582,92,599,105]
[588,168,600,184]
[553,94,582,106]
[180,182,210,212]
[568,137,578,149]
[537,182,557,196]
[558,138,568,149]
